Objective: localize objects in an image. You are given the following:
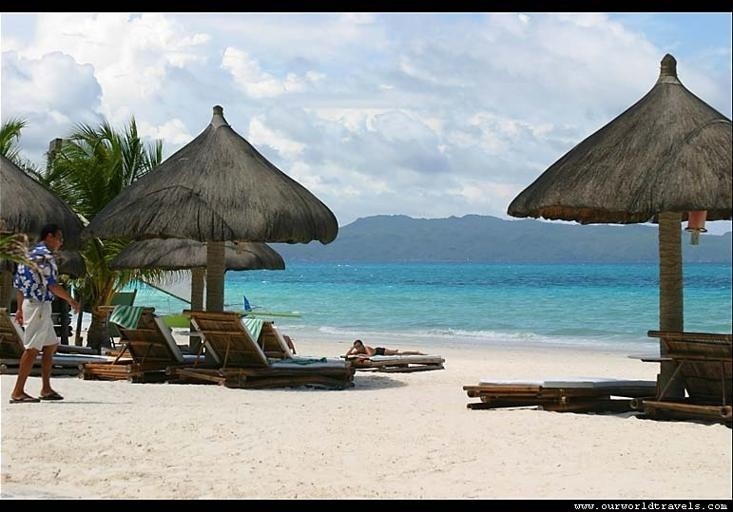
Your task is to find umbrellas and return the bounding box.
[507,53,732,399]
[0,251,86,312]
[88,105,338,311]
[0,153,87,306]
[108,240,285,354]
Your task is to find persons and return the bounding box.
[9,224,82,404]
[347,340,424,356]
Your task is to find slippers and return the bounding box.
[39,393,64,401]
[9,397,41,403]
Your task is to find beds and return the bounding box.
[461,377,657,419]
[346,356,448,373]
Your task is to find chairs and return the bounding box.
[0,289,200,383]
[173,310,354,391]
[638,328,731,425]
